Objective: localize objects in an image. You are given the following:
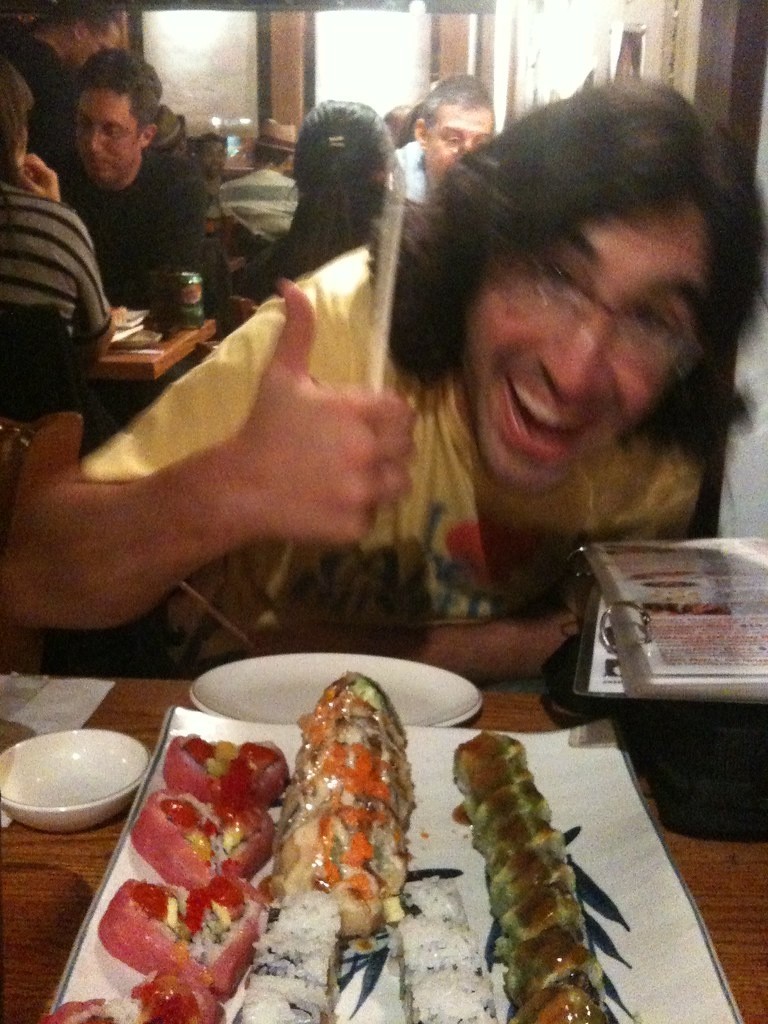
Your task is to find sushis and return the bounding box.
[169,876,258,1002]
[130,787,219,887]
[40,999,136,1024]
[235,670,414,1024]
[98,879,186,977]
[453,736,610,1024]
[212,796,275,881]
[163,736,219,799]
[132,975,228,1024]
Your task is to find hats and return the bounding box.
[146,106,189,153]
[255,119,301,156]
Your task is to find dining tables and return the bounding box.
[0,674,767,1024]
[99,308,215,381]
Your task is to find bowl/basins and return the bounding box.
[0,728,149,834]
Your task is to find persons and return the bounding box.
[0,59,123,465]
[204,135,301,244]
[176,131,229,237]
[0,76,768,688]
[56,48,216,342]
[382,72,496,206]
[0,1,124,211]
[232,100,400,307]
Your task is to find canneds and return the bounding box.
[174,271,205,330]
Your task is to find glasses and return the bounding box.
[517,238,703,369]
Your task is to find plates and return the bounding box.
[111,306,150,328]
[114,330,162,347]
[43,708,746,1024]
[190,650,484,728]
